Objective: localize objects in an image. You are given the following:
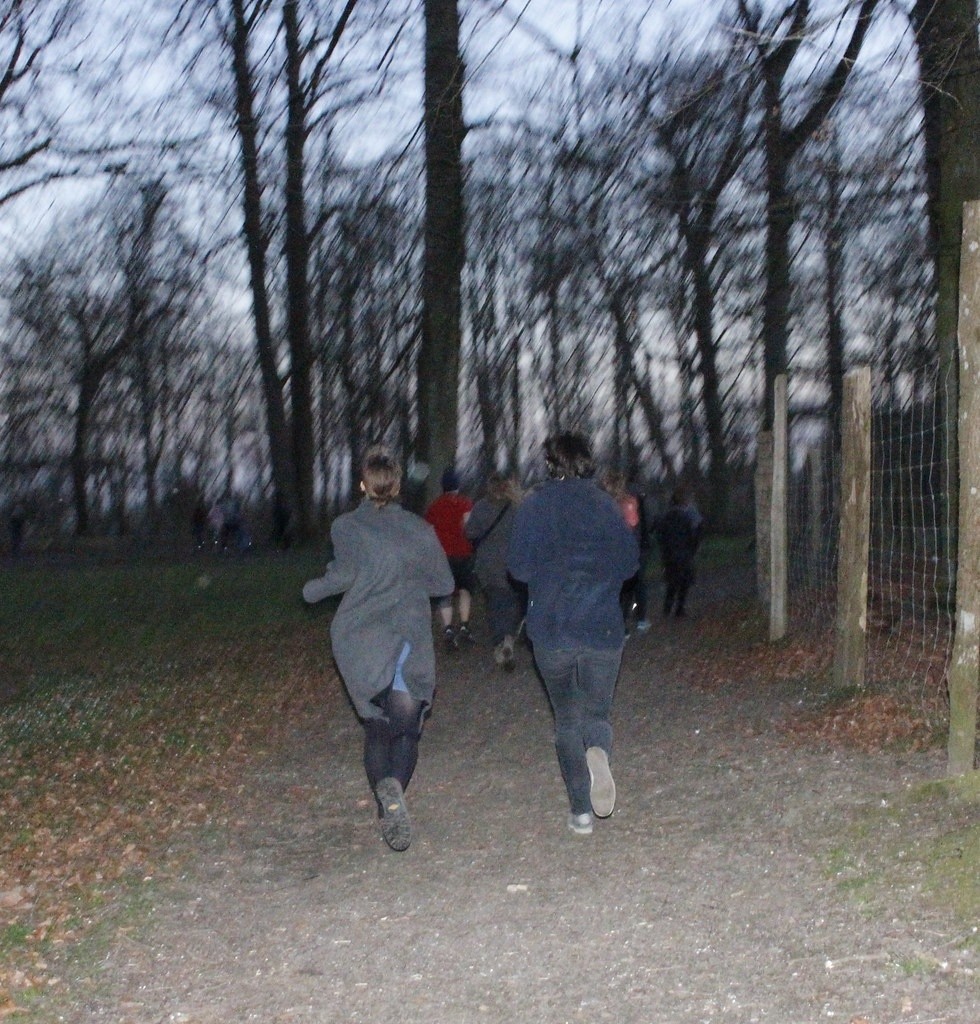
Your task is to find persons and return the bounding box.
[303,451,459,854]
[506,427,644,836]
[420,458,705,647]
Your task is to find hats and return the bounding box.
[442,468,460,491]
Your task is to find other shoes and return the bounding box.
[503,634,516,672]
[625,634,631,640]
[461,626,476,643]
[585,746,616,817]
[375,777,412,850]
[444,628,458,648]
[637,621,651,629]
[494,641,504,664]
[569,813,594,834]
